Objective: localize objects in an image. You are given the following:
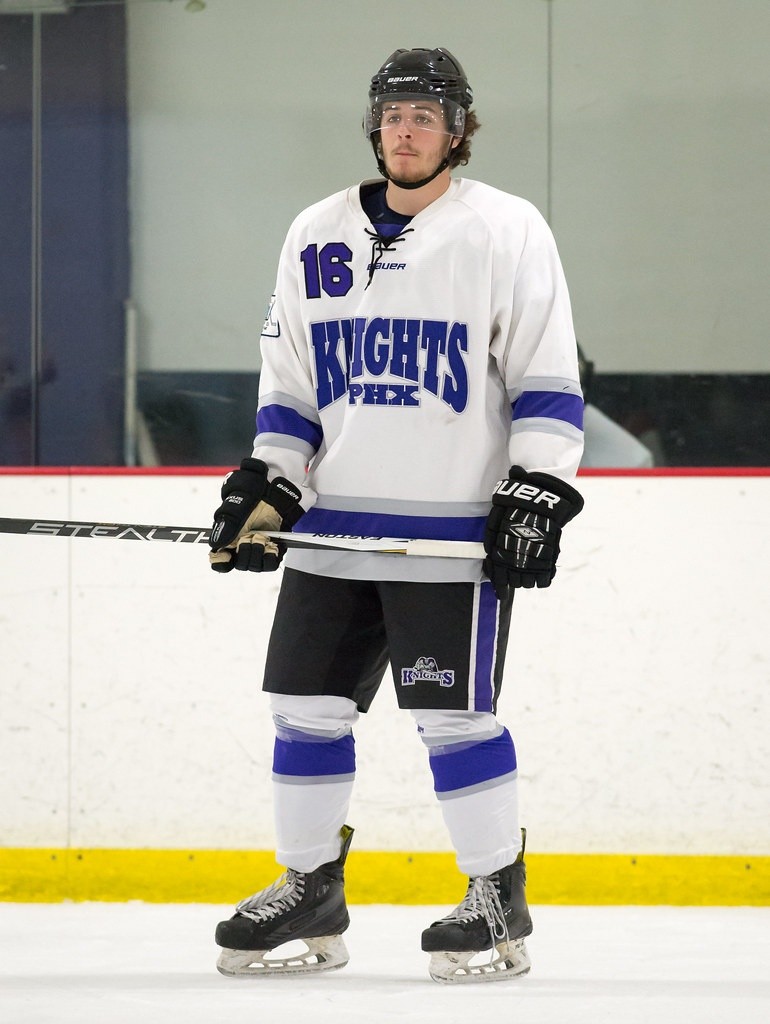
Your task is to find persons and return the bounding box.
[207,48,587,958]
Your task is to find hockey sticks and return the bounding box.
[0,516,490,563]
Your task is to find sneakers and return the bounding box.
[215,824,355,977]
[421,828,533,985]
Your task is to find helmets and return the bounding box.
[364,47,473,141]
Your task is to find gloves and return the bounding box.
[209,458,306,573]
[483,466,585,600]
